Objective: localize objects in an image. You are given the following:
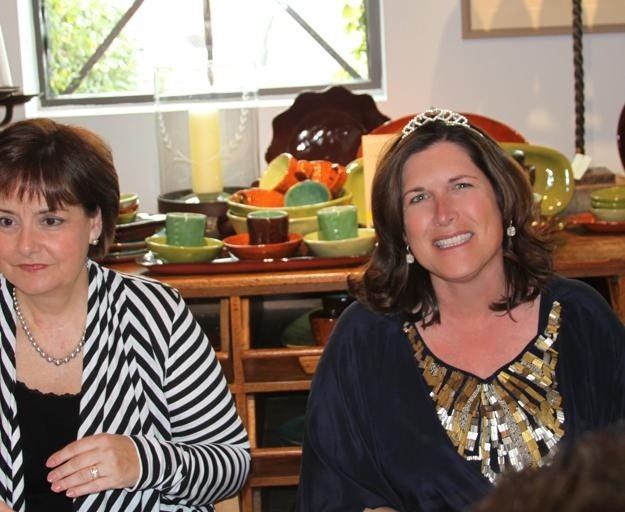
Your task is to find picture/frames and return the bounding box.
[462,1,625,39]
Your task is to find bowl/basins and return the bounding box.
[309,309,337,347]
[322,295,357,318]
[143,187,376,265]
[116,190,140,224]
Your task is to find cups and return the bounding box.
[166,152,358,248]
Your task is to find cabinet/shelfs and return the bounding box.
[100,175,625,512]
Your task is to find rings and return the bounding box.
[88,464,100,480]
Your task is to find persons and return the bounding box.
[0,115,255,512]
[290,104,624,511]
[472,420,625,512]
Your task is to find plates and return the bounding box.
[355,112,529,159]
[91,219,166,264]
[276,414,307,446]
[565,186,625,235]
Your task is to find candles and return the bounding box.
[188,107,224,200]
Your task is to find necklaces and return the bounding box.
[12,286,89,366]
